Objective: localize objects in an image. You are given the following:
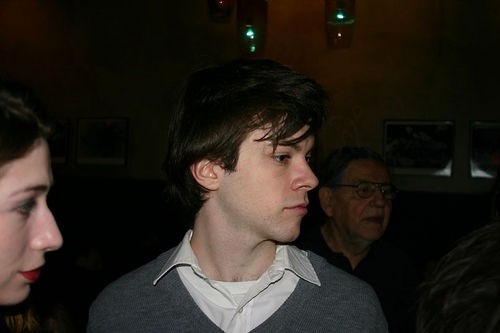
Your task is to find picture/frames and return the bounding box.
[381,119,455,178]
[75,116,130,168]
[468,120,500,181]
[48,118,72,165]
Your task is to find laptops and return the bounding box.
[383,120,500,193]
[40,116,131,176]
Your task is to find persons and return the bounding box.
[484,144,500,224]
[410,219,500,333]
[86,56,391,333]
[0,80,71,333]
[297,145,405,333]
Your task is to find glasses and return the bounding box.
[329,181,398,200]
